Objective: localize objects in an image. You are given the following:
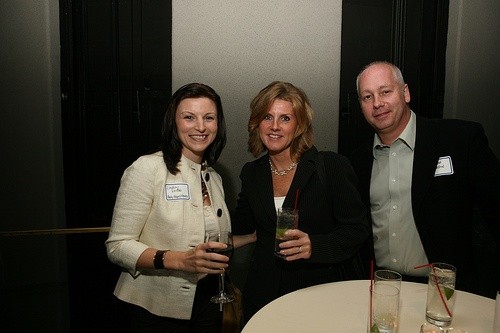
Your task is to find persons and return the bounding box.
[105,84,257,333]
[351,61,500,300]
[228,82,373,324]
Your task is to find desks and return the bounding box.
[240,280,500,333]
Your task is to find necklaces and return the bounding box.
[269,159,298,175]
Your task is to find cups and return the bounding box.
[426,263,456,326]
[420,323,453,333]
[374,270,402,325]
[493,292,500,333]
[368,283,399,333]
[274,209,298,260]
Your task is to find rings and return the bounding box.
[299,246,302,253]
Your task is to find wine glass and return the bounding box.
[208,231,234,304]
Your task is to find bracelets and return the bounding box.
[153,249,170,271]
